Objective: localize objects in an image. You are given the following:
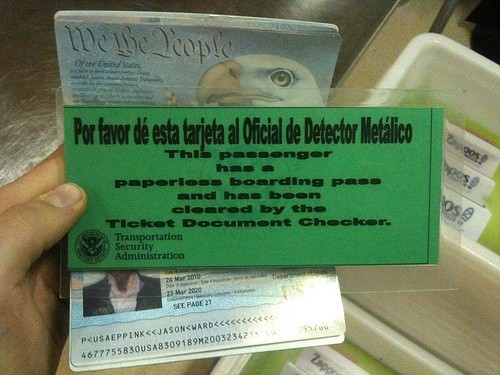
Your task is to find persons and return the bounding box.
[1,138,87,374]
[80,270,163,318]
